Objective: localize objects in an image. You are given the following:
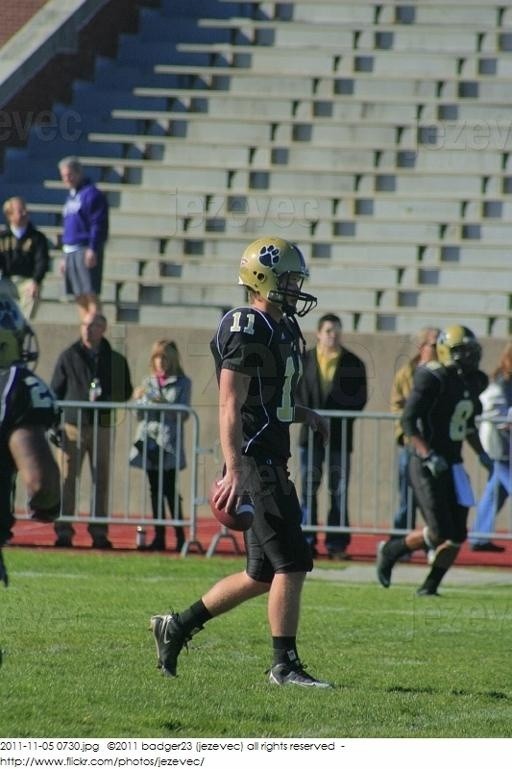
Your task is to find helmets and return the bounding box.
[0,290,38,366]
[238,237,317,316]
[437,325,480,367]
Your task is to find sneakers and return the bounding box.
[149,614,192,676]
[377,540,392,587]
[265,657,329,688]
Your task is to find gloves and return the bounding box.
[293,406,326,435]
[420,448,448,478]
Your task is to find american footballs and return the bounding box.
[210,477,254,531]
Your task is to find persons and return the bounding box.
[390,326,447,562]
[149,235,335,689]
[1,281,61,666]
[291,310,367,560]
[57,156,109,346]
[468,343,511,554]
[0,197,48,327]
[375,324,493,600]
[130,338,193,554]
[51,313,133,549]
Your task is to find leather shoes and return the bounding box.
[473,543,504,552]
